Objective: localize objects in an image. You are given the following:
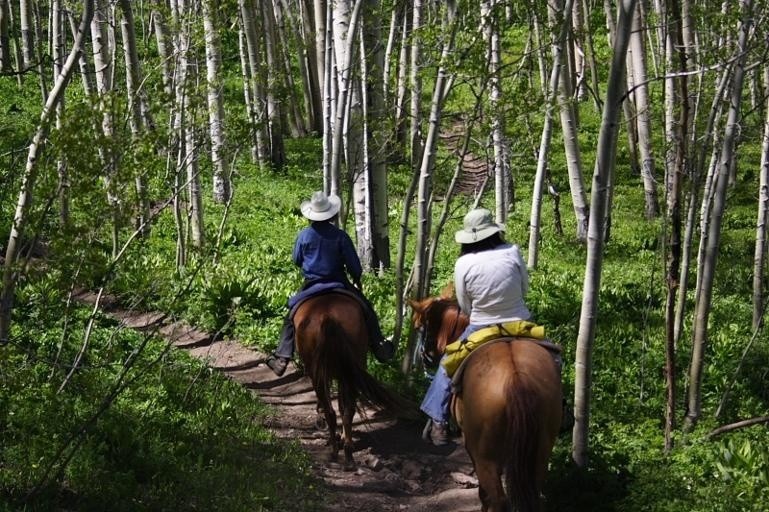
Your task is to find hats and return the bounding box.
[453,209,507,244]
[299,188,342,223]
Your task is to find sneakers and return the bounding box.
[374,338,395,364]
[264,354,288,377]
[429,418,451,447]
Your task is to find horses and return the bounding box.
[403,279,562,512]
[292,293,391,470]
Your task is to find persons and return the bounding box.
[263,191,394,377]
[421,208,575,446]
